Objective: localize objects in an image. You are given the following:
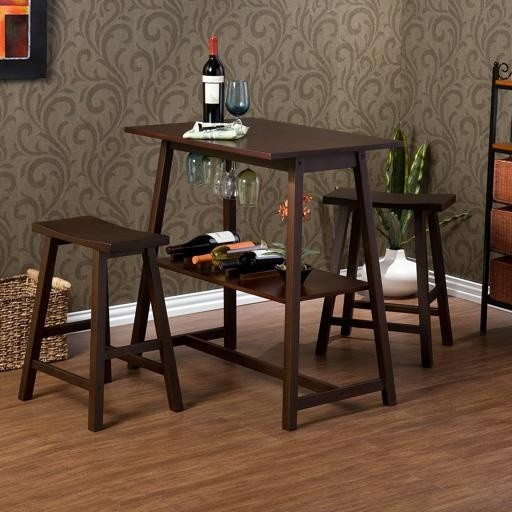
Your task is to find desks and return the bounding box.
[119,117,404,432]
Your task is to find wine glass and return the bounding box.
[225,79,249,126]
[186,151,260,207]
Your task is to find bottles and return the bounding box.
[167,229,284,273]
[202,32,226,123]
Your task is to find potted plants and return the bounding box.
[355,119,472,301]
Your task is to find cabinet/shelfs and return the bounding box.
[479,63,512,336]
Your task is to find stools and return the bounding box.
[16,215,186,432]
[314,187,456,368]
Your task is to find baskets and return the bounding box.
[489,206,512,257]
[489,256,512,305]
[491,157,512,205]
[0,268,72,371]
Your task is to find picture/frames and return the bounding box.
[0,0,49,79]
